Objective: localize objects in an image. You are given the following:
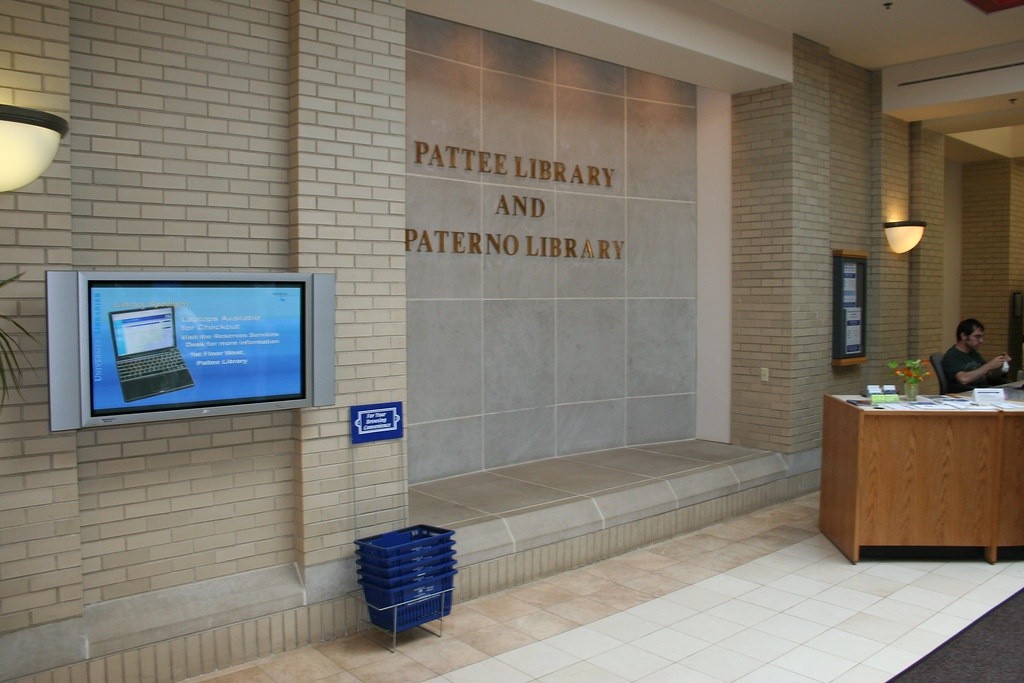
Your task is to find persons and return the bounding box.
[940,319,1012,394]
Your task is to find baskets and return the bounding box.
[355,525,458,635]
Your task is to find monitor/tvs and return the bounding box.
[45,269,336,431]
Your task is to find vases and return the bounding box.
[904,382,920,401]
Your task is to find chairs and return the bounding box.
[930,352,949,395]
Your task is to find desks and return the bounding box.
[819,394,1024,565]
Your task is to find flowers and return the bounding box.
[888,359,931,383]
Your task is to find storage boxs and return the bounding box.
[354,524,459,633]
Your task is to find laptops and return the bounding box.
[108,305,195,402]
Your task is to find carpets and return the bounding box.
[886,587,1024,683]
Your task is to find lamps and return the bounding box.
[0,103,68,193]
[883,220,927,255]
